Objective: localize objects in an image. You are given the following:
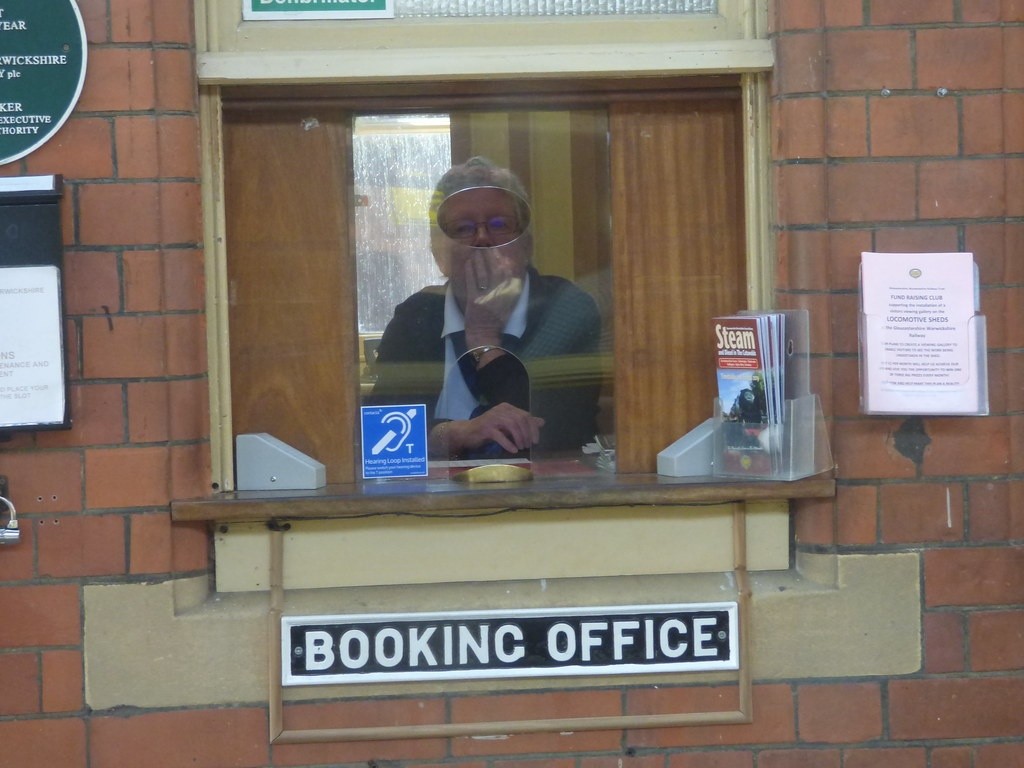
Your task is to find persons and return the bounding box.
[365,158,602,460]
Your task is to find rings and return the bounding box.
[479,286,488,289]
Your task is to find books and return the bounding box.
[711,313,787,476]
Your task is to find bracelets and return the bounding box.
[474,343,505,362]
[438,420,459,459]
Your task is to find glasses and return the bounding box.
[447,215,524,239]
